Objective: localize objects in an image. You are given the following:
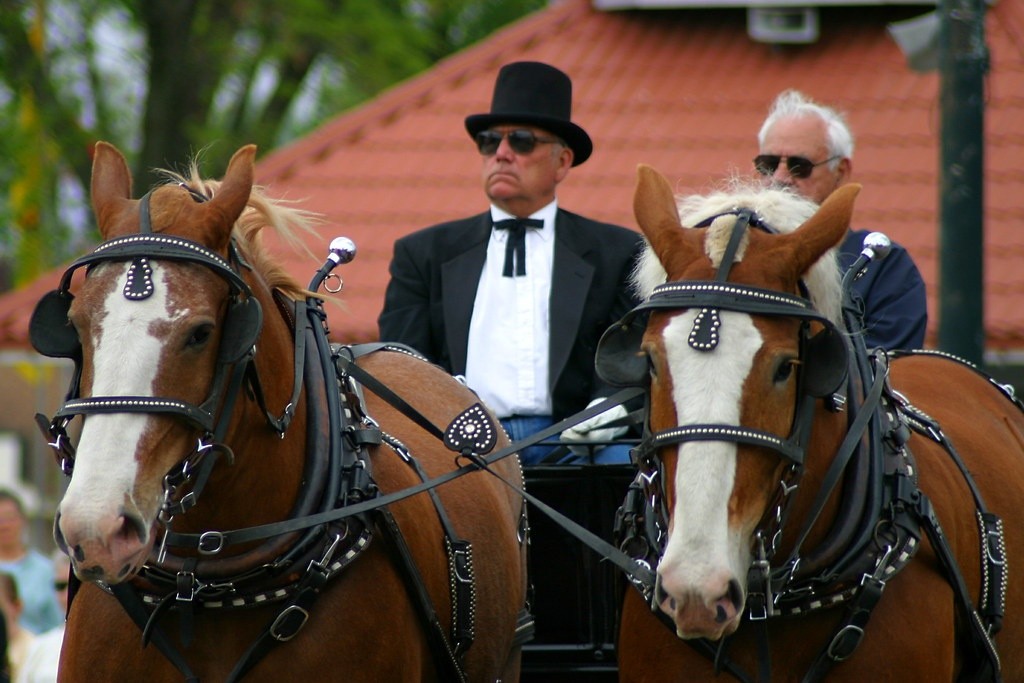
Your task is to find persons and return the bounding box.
[377,60,651,464]
[753,89,929,359]
[0,489,71,683]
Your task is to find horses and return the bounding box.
[53,140,529,683]
[617,165,1024,683]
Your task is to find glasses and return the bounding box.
[753,153,846,178]
[476,129,566,155]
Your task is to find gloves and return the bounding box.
[561,397,630,455]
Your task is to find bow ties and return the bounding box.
[494,218,544,277]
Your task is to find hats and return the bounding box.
[464,61,593,168]
